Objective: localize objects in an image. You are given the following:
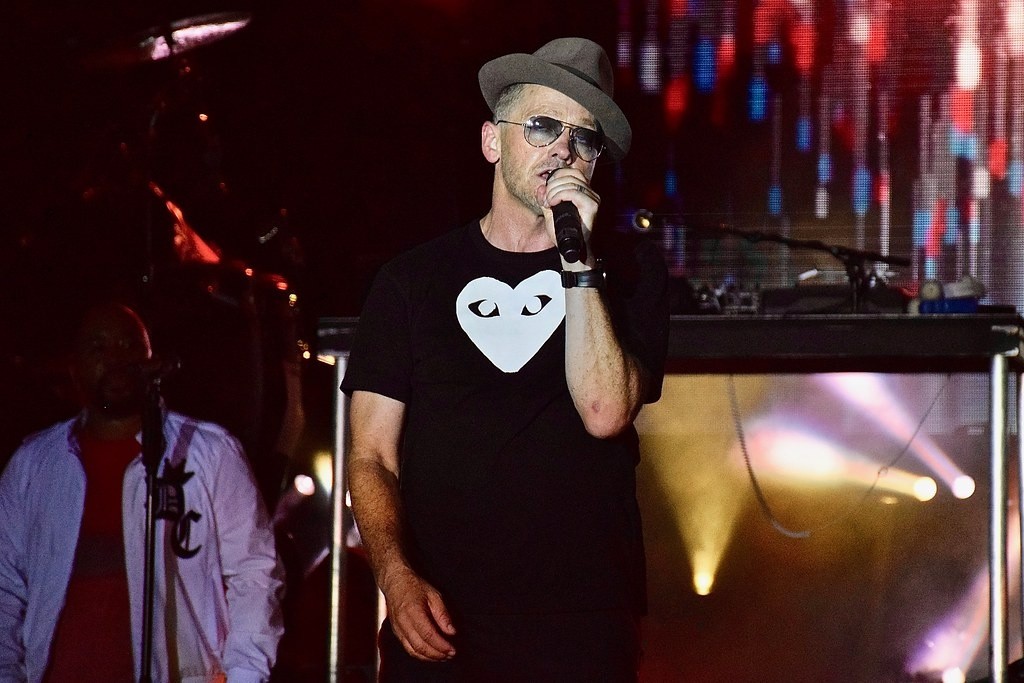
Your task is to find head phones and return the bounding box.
[918,277,984,299]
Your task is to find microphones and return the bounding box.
[547,168,583,264]
[631,209,654,232]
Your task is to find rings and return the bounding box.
[576,185,587,193]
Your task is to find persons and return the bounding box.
[340,37,670,683]
[0,301,287,683]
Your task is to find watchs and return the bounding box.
[562,268,606,289]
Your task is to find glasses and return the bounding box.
[495,116,606,162]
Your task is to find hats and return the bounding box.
[478,38,631,165]
